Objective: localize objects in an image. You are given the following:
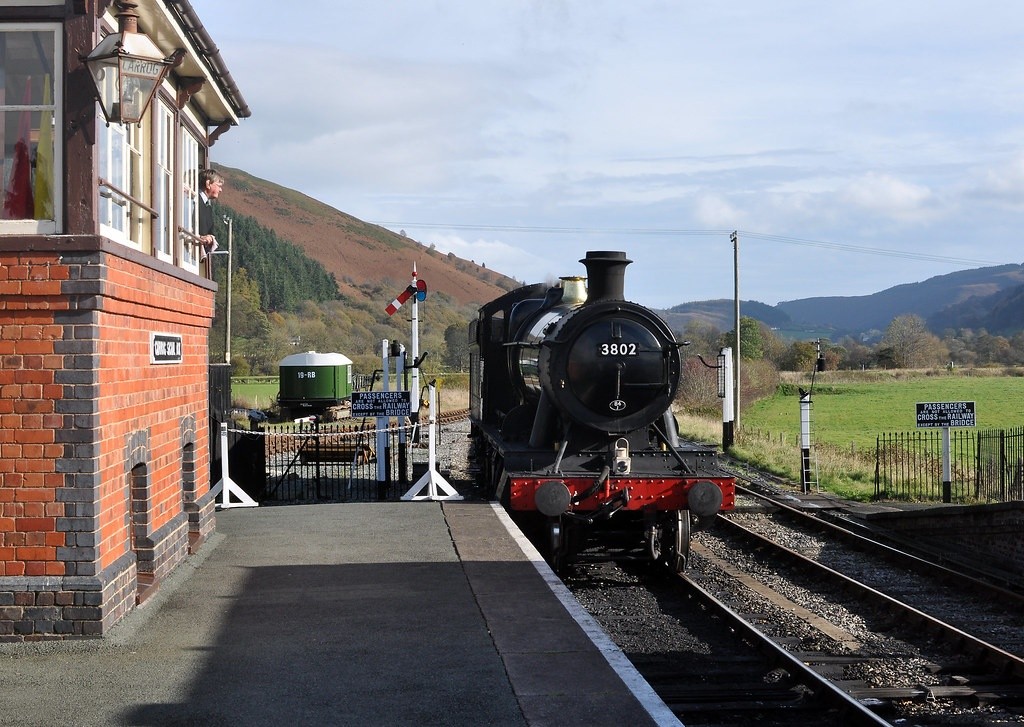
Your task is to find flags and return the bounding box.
[33,76,55,221]
[1,79,34,220]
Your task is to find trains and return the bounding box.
[466,251,737,576]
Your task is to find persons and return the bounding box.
[191,170,225,253]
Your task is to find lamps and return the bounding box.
[73,0,175,130]
